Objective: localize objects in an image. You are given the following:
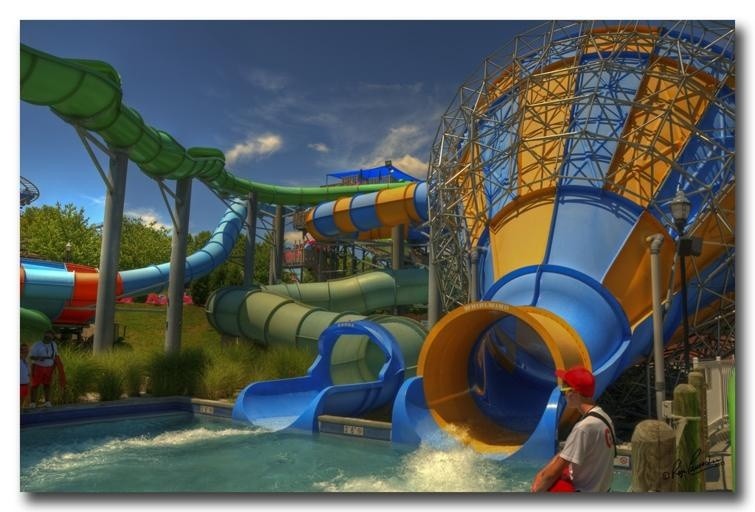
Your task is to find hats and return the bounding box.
[555,367,595,397]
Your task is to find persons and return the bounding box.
[21,329,64,414]
[529,366,616,493]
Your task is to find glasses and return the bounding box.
[560,387,572,396]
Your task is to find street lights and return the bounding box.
[64,239,74,263]
[668,182,705,381]
